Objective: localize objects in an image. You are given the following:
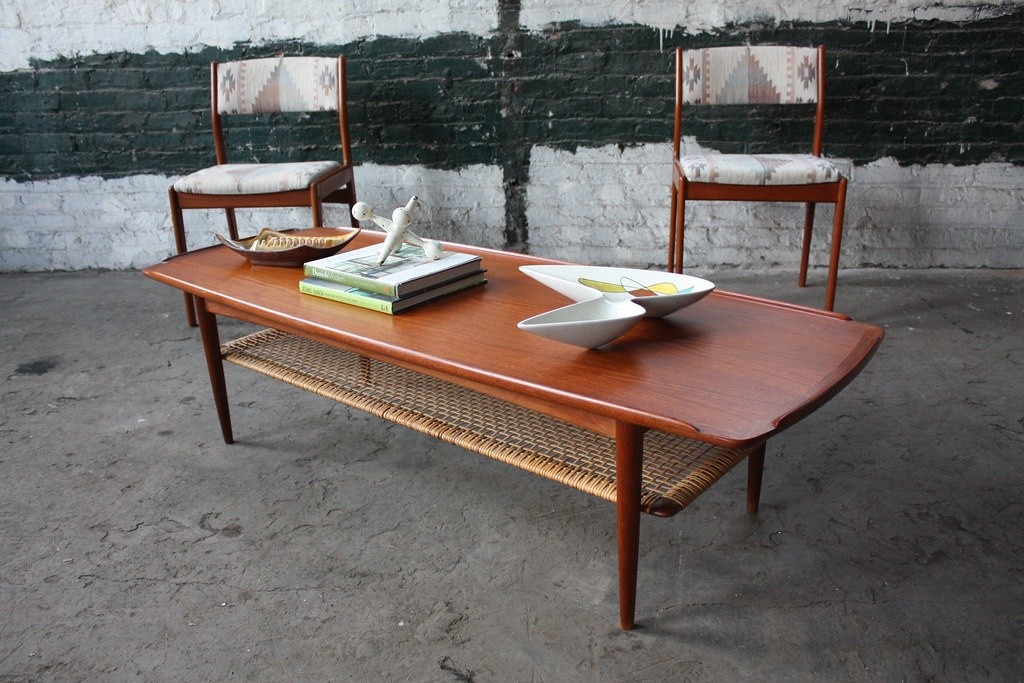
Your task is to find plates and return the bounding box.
[216,227,360,266]
[514,264,715,350]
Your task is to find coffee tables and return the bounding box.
[141,224,886,633]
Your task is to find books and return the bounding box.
[299,240,488,315]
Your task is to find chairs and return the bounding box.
[167,53,362,328]
[668,44,849,313]
